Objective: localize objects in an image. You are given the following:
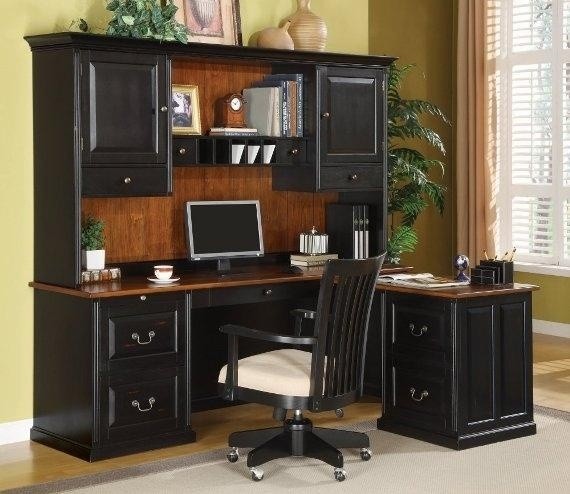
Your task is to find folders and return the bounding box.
[325,203,369,260]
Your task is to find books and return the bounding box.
[376,272,470,290]
[207,72,304,138]
[290,260,329,268]
[290,253,339,260]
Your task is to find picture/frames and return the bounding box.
[172,83,202,135]
[160,0,244,45]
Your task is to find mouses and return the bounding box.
[285,265,302,274]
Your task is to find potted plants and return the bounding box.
[80,212,107,269]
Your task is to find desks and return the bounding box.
[29,264,542,463]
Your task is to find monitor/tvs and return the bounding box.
[184,201,265,272]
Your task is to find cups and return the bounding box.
[152,264,174,279]
[232,144,277,165]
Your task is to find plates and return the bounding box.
[146,276,181,284]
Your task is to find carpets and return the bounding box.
[0,403,570,494]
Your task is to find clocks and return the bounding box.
[220,92,248,127]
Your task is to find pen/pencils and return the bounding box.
[494,246,516,262]
[483,249,489,261]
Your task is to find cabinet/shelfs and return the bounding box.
[23,30,172,197]
[168,42,313,169]
[271,48,397,191]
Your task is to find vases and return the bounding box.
[280,0,328,51]
[257,17,296,50]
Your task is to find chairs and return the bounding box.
[213,250,389,483]
